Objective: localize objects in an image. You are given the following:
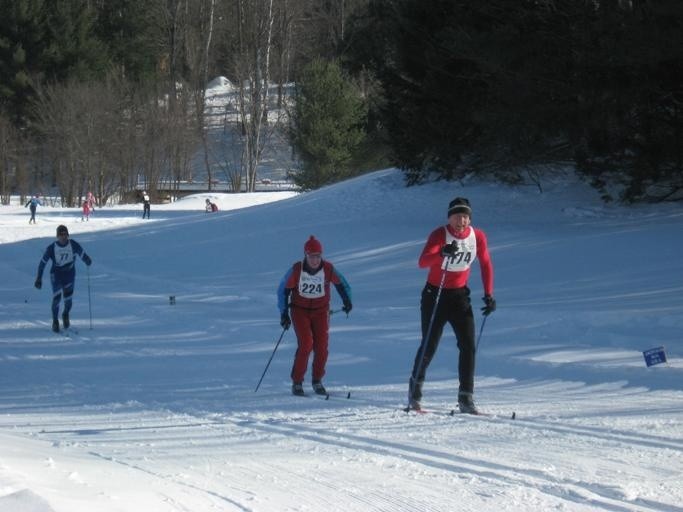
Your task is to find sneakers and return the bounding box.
[292,383,305,396]
[62,311,70,329]
[52,319,59,333]
[312,381,327,395]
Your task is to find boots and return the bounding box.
[408,374,423,410]
[457,390,481,416]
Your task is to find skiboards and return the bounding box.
[269,380,352,400]
[375,397,517,419]
[35,314,78,338]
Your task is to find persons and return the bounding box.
[278,233,352,397]
[33,225,91,333]
[407,196,495,414]
[81,192,95,222]
[203,198,217,211]
[23,192,42,225]
[140,189,151,220]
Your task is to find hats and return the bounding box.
[447,197,472,220]
[56,225,68,236]
[304,235,322,256]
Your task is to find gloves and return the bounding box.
[280,311,291,330]
[342,297,352,314]
[85,258,92,266]
[440,239,460,258]
[479,294,496,317]
[34,280,42,290]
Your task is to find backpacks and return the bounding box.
[31,199,37,207]
